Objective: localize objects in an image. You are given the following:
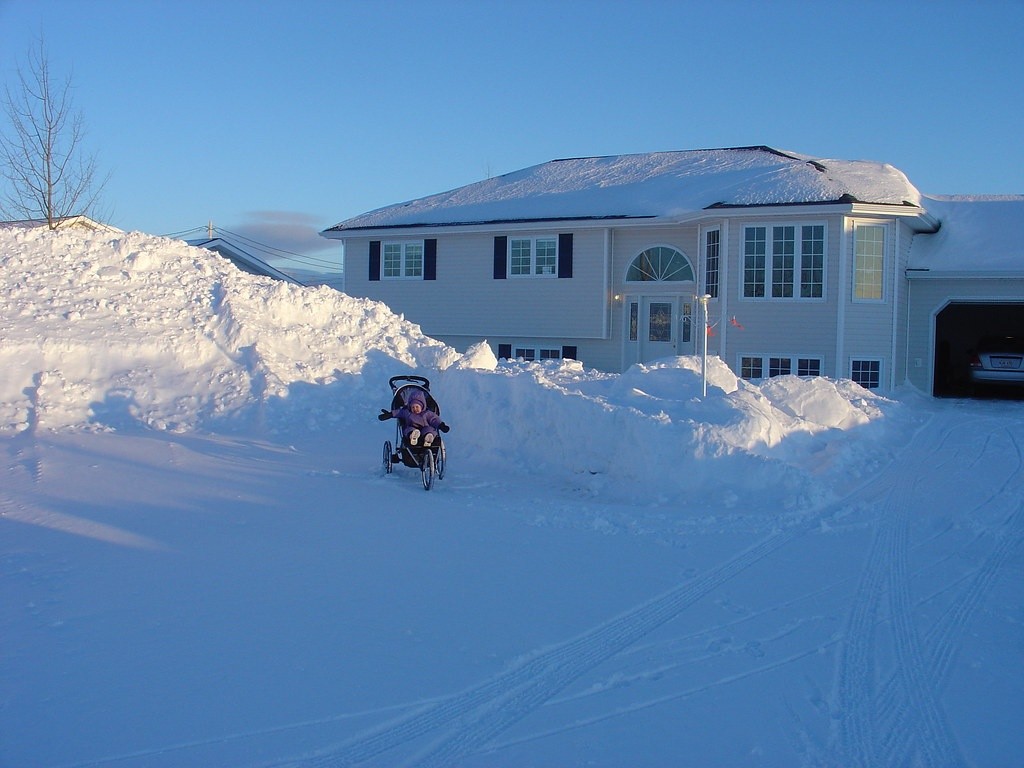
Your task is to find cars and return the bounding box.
[969,330,1024,391]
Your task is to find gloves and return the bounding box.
[378,409,393,421]
[438,422,450,433]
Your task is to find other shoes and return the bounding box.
[423,433,434,448]
[410,429,421,445]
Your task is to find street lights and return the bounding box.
[699,293,711,397]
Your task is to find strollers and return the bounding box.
[384,376,446,490]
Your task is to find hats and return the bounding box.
[410,400,423,409]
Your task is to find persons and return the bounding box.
[378,391,450,447]
